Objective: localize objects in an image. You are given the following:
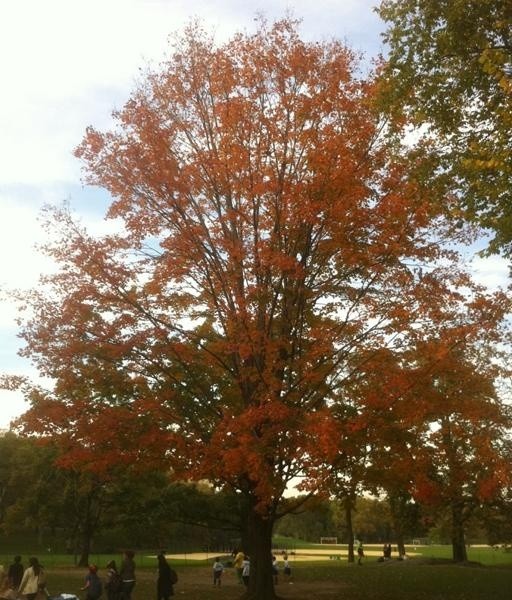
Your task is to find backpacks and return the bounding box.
[108,570,122,594]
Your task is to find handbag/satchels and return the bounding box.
[170,569,179,585]
[37,570,47,590]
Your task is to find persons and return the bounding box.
[157,554,173,600]
[272,556,279,582]
[80,564,101,600]
[212,557,224,585]
[383,544,388,560]
[104,560,119,600]
[387,545,391,559]
[7,556,51,600]
[233,549,245,584]
[241,557,250,586]
[120,550,136,600]
[357,543,365,564]
[283,557,289,581]
